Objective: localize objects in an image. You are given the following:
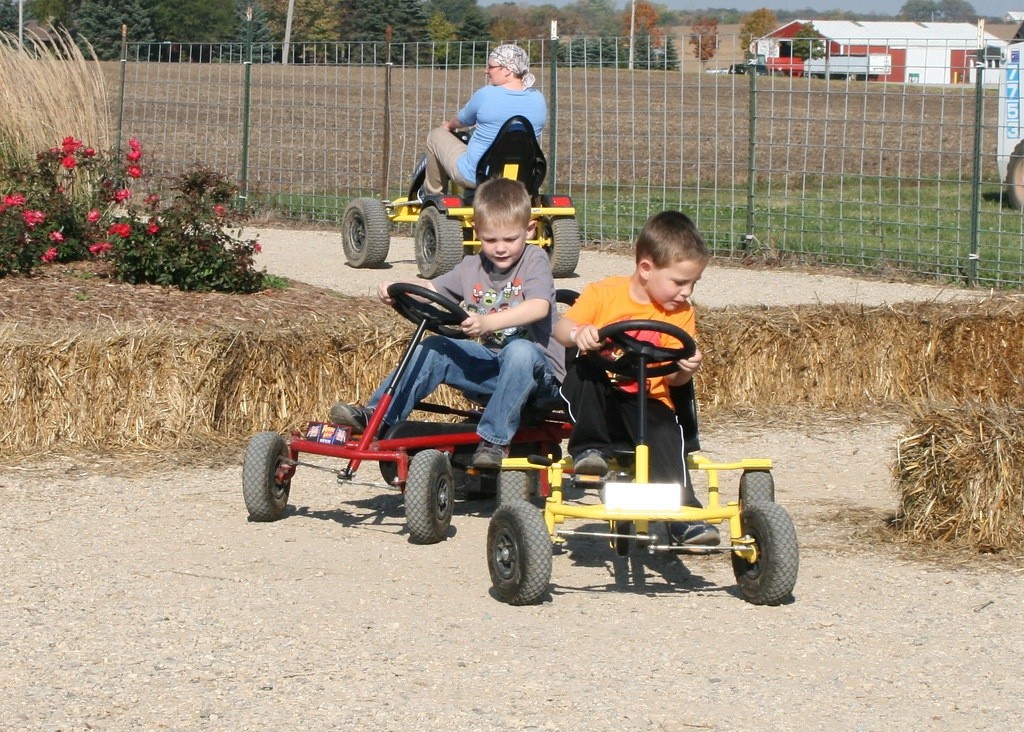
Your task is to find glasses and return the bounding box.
[487,64,504,70]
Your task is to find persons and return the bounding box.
[417,44,547,204]
[330,178,567,468]
[553,211,721,546]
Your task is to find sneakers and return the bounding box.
[669,522,720,546]
[574,448,609,477]
[471,437,511,467]
[417,186,439,204]
[330,403,393,441]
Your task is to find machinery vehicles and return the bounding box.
[728,54,892,82]
